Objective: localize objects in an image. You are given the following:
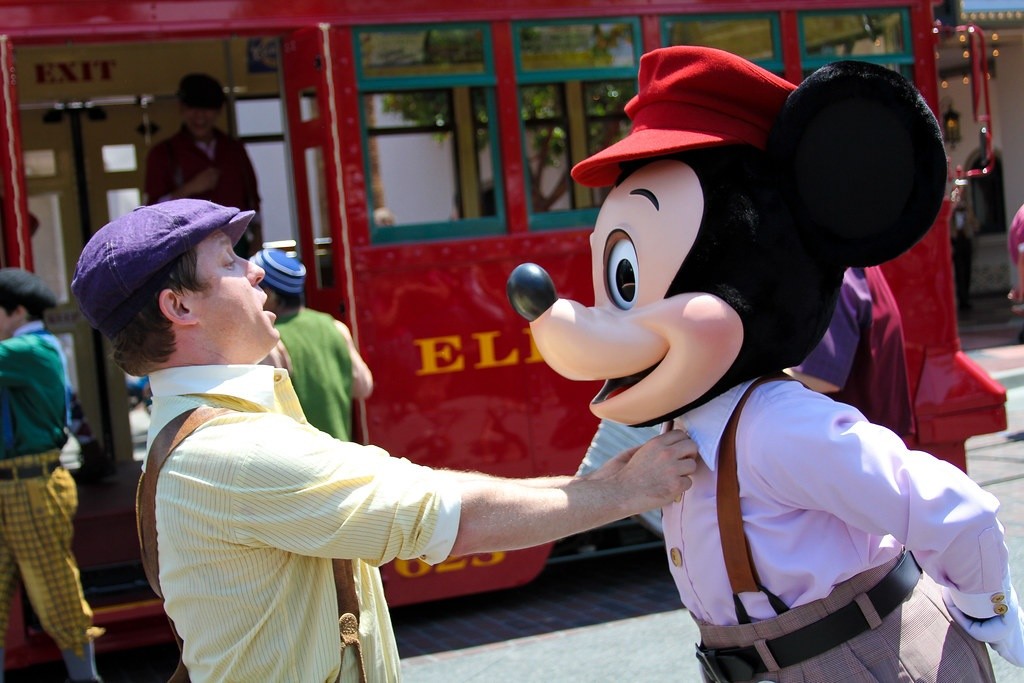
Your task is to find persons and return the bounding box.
[145,73,261,263]
[778,182,1024,438]
[69,200,697,683]
[0,268,105,683]
[247,248,373,442]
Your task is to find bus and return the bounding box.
[1,0,1006,670]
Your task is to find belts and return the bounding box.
[0,461,61,481]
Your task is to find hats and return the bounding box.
[251,250,305,294]
[177,73,224,112]
[71,199,256,338]
[0,269,56,313]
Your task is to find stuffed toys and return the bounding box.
[508,43,1024,683]
